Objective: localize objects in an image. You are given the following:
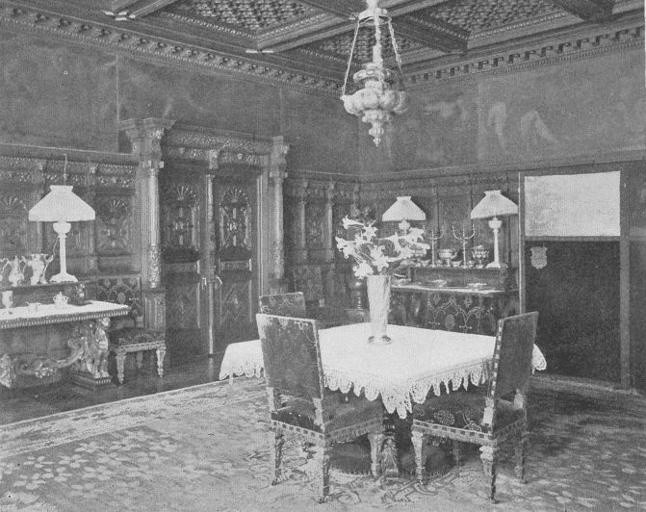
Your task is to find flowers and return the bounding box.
[334,214,430,279]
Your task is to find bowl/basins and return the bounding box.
[467,282,488,291]
[431,280,447,289]
[437,248,453,260]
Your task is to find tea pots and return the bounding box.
[52,291,69,311]
[0,252,55,288]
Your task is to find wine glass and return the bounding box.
[470,246,490,269]
[2,291,13,314]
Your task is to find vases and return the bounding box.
[365,274,392,346]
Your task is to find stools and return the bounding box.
[107,326,167,384]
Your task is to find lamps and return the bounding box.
[27,183,97,282]
[381,195,428,234]
[339,0,412,149]
[469,188,521,271]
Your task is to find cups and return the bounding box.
[27,302,39,316]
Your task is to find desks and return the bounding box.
[229,320,537,483]
[0,298,132,395]
